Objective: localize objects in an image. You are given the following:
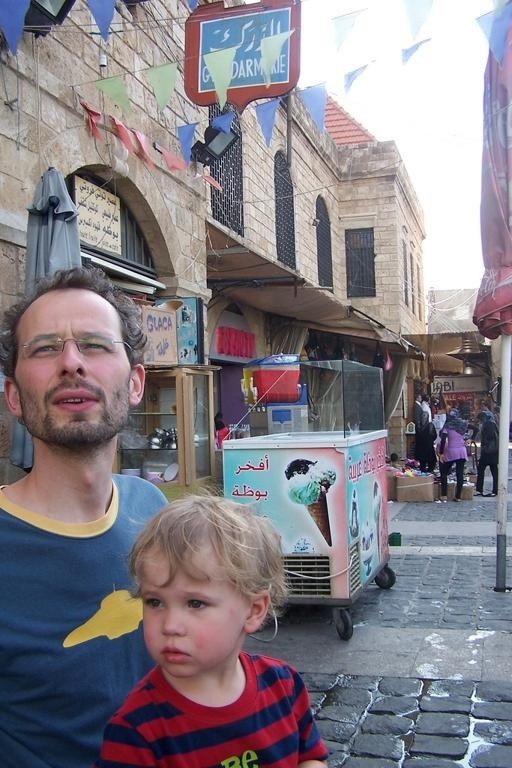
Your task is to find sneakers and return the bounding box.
[473,491,496,497]
[440,496,461,502]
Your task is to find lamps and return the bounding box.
[191,125,239,167]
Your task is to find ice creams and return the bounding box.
[284,458,336,546]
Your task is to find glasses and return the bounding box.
[16,335,133,354]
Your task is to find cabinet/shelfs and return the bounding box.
[113,368,216,502]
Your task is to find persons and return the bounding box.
[100,485,331,766]
[434,407,468,502]
[435,429,452,475]
[390,454,401,468]
[421,394,433,422]
[413,393,423,433]
[1,264,172,768]
[413,409,437,472]
[475,411,499,497]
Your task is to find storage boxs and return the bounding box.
[389,474,475,502]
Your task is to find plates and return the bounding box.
[164,462,179,484]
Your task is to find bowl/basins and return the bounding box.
[146,470,161,480]
[120,468,142,477]
